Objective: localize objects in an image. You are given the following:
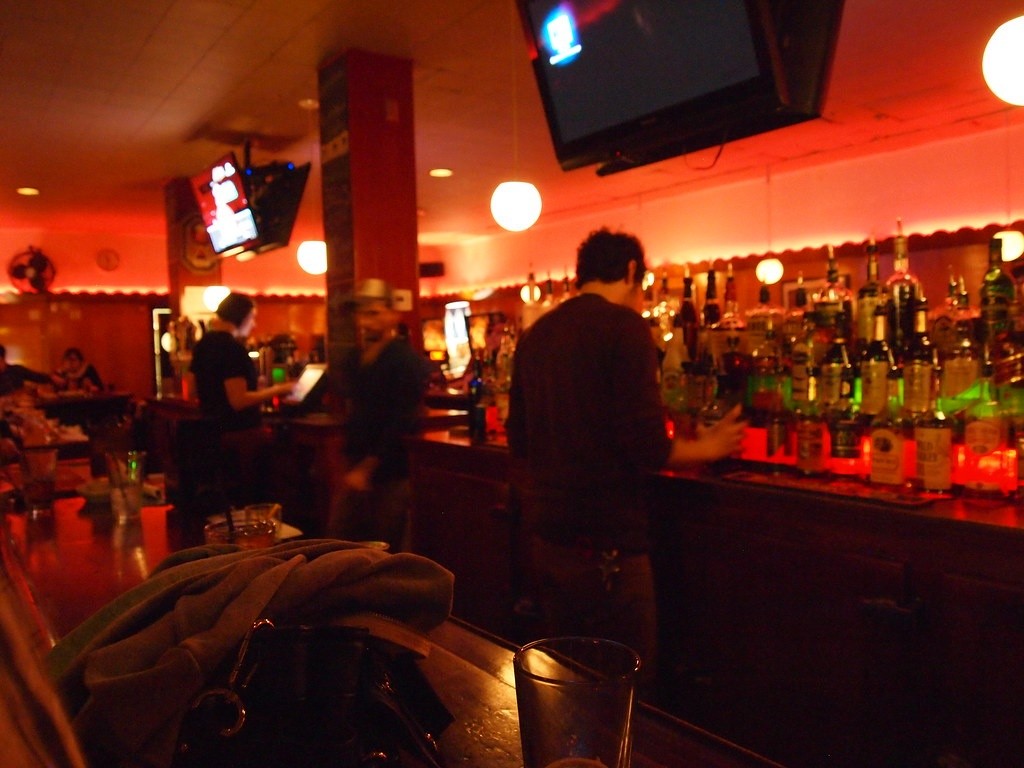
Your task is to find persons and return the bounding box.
[325,302,425,553]
[194,294,291,495]
[64,347,103,391]
[0,344,66,398]
[509,232,744,705]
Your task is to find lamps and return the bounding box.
[296,241,328,275]
[489,0,541,231]
[981,16,1024,106]
[204,285,231,311]
[755,151,784,285]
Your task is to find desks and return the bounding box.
[0,387,775,768]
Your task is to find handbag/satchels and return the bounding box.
[173,614,455,768]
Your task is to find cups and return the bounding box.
[513,636,642,768]
[204,520,274,552]
[107,452,145,524]
[245,503,281,537]
[25,450,57,494]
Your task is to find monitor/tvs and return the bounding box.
[283,364,329,405]
[190,152,311,261]
[515,0,846,176]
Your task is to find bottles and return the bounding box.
[982,238,1013,338]
[858,245,889,361]
[860,305,895,426]
[823,312,854,416]
[886,235,921,353]
[915,364,952,499]
[963,361,1002,508]
[520,270,808,377]
[796,367,823,467]
[902,296,938,425]
[748,331,780,425]
[867,370,904,495]
[830,364,861,473]
[768,366,786,457]
[468,356,483,407]
[792,320,817,402]
[817,257,851,347]
